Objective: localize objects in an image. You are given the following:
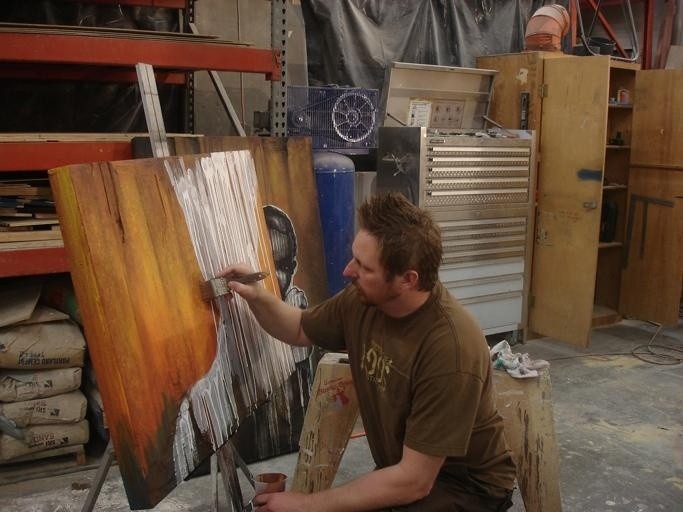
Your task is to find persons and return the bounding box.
[212,189,517,511]
[261,203,313,364]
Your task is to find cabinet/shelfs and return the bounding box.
[474,53,683,351]
[0,22,289,281]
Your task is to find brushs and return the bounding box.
[200,272,271,302]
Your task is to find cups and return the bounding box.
[250,471,289,507]
